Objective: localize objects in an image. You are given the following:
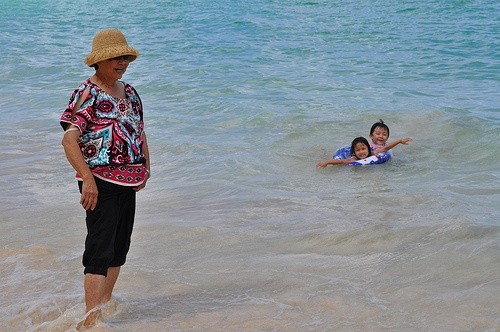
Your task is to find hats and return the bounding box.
[85,28,138,67]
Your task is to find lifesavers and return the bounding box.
[332,142,392,166]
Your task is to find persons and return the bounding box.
[59,30,151,323]
[368,118,394,157]
[314,136,413,169]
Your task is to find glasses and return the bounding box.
[112,55,130,60]
